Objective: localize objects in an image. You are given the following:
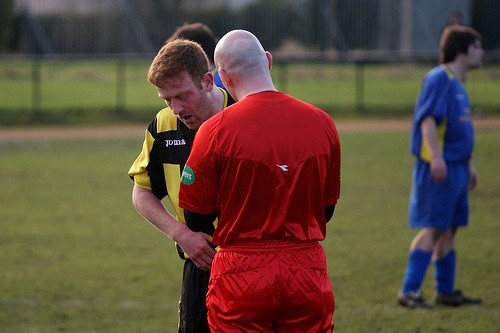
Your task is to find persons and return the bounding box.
[127,40,238,333]
[165,24,236,107]
[179,30,341,333]
[396,25,485,309]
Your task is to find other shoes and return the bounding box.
[435,290,482,307]
[396,289,436,314]
